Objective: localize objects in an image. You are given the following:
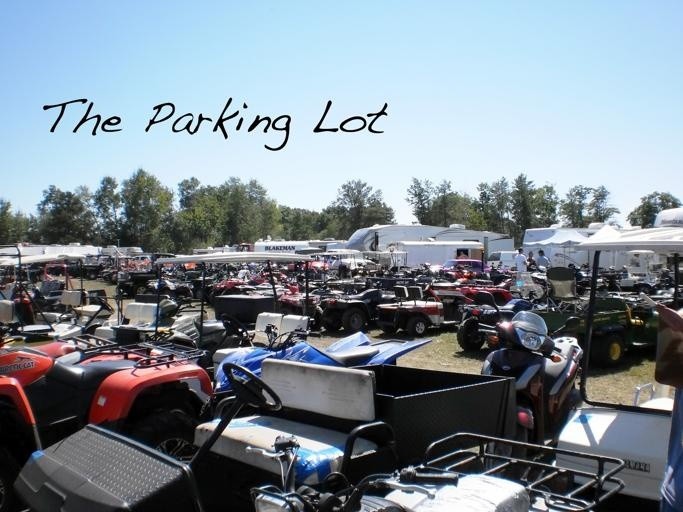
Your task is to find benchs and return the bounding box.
[193,357,380,487]
[94,303,158,344]
[34,289,83,324]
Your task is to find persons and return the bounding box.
[537,249,549,272]
[514,247,527,271]
[490,262,496,268]
[651,304,683,512]
[498,261,502,268]
[527,251,537,271]
[456,251,469,259]
